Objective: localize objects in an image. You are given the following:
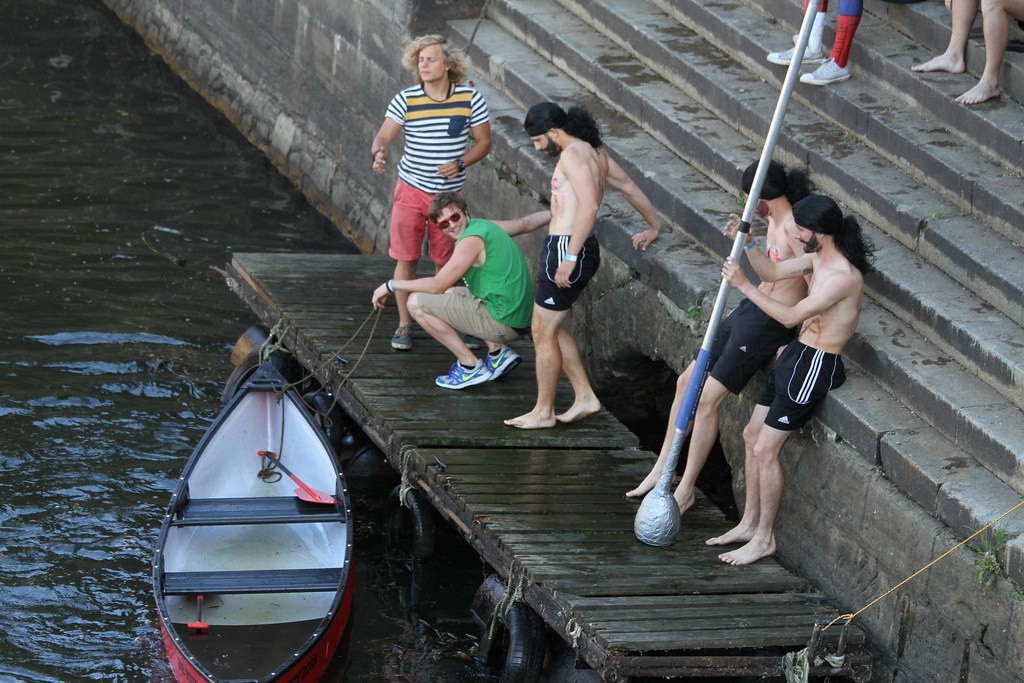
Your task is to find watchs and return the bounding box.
[455,158,466,172]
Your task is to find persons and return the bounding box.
[911,0,1024,106]
[502,100,660,430]
[370,35,492,350]
[372,190,552,390]
[626,159,814,516]
[767,0,864,85]
[706,193,864,566]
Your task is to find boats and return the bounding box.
[152,357,355,683]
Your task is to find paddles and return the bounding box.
[258,449,340,506]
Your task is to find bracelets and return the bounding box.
[743,240,756,249]
[563,253,578,263]
[385,279,395,292]
[372,149,381,162]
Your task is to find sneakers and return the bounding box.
[485,344,522,381]
[435,359,492,389]
[391,327,413,350]
[767,34,825,65]
[462,332,483,349]
[799,58,851,86]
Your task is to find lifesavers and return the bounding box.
[478,600,552,681]
[381,484,438,583]
[220,348,302,405]
[303,392,345,446]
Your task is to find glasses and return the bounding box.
[435,208,463,230]
